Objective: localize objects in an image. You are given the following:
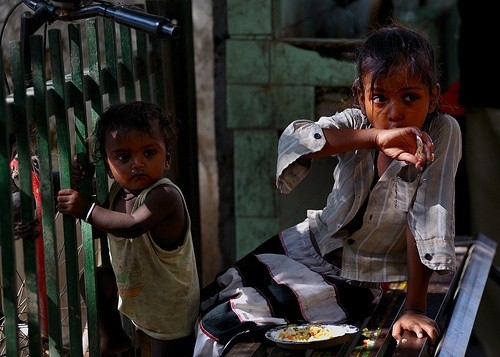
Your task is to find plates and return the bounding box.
[265,324,359,349]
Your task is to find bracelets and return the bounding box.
[85,203,96,223]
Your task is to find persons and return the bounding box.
[194,26,463,357]
[56,101,200,357]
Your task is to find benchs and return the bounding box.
[226,236,474,357]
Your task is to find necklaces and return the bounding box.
[120,193,140,201]
